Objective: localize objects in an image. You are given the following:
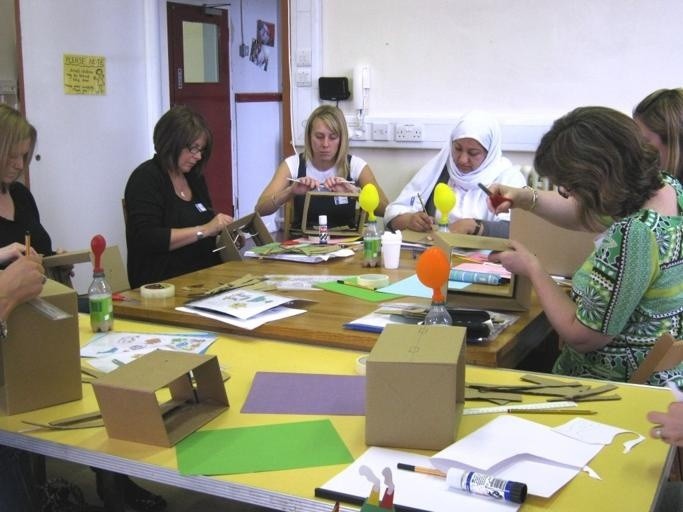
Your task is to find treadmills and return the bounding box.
[463,401,577,416]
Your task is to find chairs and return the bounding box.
[96,478,168,512]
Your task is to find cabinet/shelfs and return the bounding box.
[87,269,114,334]
[435,220,452,236]
[361,219,383,268]
[423,294,453,329]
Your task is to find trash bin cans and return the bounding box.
[0,318,8,339]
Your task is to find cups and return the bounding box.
[187,143,207,155]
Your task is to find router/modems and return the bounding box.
[140,282,175,299]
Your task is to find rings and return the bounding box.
[337,280,378,291]
[416,193,434,232]
[286,177,355,188]
[507,409,597,415]
[25,231,30,257]
[397,463,448,477]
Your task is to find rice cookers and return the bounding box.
[426,310,490,339]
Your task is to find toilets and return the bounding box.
[352,58,369,111]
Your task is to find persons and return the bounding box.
[383,110,527,238]
[124,104,245,290]
[0,241,43,512]
[485,106,683,390]
[0,103,167,511]
[254,104,389,243]
[647,401,683,448]
[632,88,683,185]
[249,21,270,72]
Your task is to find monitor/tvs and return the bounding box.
[244,248,355,263]
[342,302,520,344]
[315,445,522,512]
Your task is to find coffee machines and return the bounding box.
[479,184,513,213]
[112,293,140,304]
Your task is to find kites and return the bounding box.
[450,271,511,286]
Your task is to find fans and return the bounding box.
[321,2,681,149]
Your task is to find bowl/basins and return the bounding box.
[175,177,185,198]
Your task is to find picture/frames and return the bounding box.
[0,314,681,512]
[109,236,572,368]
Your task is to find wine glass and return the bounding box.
[655,428,662,438]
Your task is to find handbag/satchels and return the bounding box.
[195,225,203,241]
[522,185,538,212]
[271,191,288,209]
[472,219,482,235]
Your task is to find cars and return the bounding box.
[381,236,400,271]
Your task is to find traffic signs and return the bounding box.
[394,125,424,142]
